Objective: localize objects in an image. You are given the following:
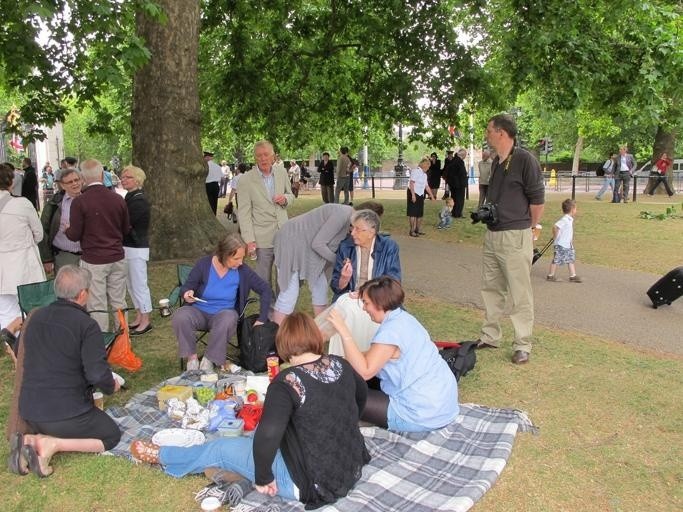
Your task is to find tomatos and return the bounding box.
[248,394,256,402]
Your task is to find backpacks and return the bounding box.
[596,159,612,176]
[239,314,284,373]
[441,340,481,382]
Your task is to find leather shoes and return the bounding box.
[475,339,495,348]
[129,321,152,336]
[512,350,529,365]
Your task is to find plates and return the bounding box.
[151,428,206,448]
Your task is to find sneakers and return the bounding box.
[547,274,561,281]
[187,357,214,371]
[569,274,582,282]
[410,230,426,237]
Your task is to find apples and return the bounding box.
[248,390,256,394]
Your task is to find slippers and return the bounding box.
[9,431,32,475]
[21,442,53,477]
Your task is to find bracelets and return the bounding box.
[531,226,536,231]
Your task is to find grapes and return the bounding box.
[196,386,215,405]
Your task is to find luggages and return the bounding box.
[647,265,683,309]
[533,238,554,264]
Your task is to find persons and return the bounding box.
[238,142,295,301]
[471,115,544,366]
[610,146,636,204]
[406,149,468,237]
[549,198,579,285]
[289,146,359,206]
[7,155,150,336]
[130,307,371,510]
[274,196,383,324]
[328,208,400,357]
[647,151,675,197]
[489,150,495,161]
[171,231,273,374]
[477,151,492,212]
[323,276,462,431]
[0,161,47,351]
[202,150,252,215]
[8,264,121,477]
[595,152,616,201]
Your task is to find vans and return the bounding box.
[630,159,682,178]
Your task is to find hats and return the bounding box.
[203,151,213,156]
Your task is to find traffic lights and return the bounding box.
[538,138,547,152]
[481,140,490,154]
[546,140,554,155]
[520,139,530,151]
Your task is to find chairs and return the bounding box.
[159,266,257,373]
[17,280,136,392]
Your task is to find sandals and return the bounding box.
[130,440,160,464]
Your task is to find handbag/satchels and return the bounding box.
[224,201,233,214]
[651,165,662,175]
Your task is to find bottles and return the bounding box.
[265,350,280,383]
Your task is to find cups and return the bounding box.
[246,243,257,260]
[92,391,104,410]
[158,298,170,317]
[534,223,542,241]
